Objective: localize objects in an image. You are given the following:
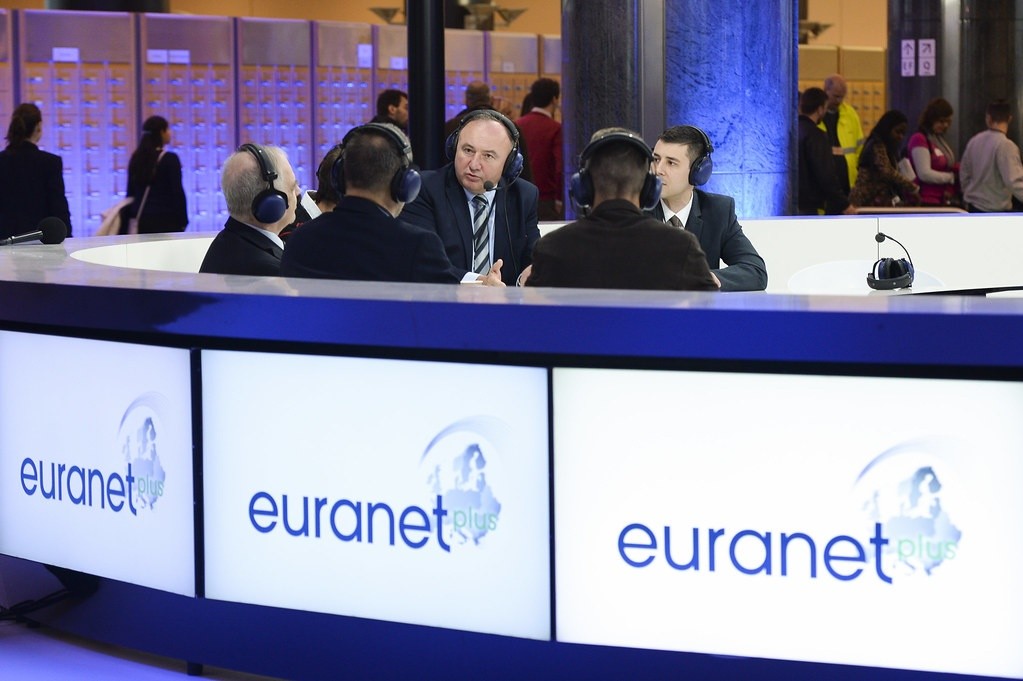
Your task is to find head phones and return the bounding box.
[445,110,525,182]
[687,125,713,186]
[332,122,421,205]
[239,143,289,224]
[569,132,662,212]
[867,258,914,290]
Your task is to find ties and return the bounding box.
[470,194,493,276]
[668,215,683,230]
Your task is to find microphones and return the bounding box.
[0,217,67,247]
[483,174,519,192]
[875,232,913,266]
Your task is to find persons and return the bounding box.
[280,117,462,284]
[447,81,532,183]
[370,88,410,138]
[909,94,961,206]
[279,141,346,242]
[957,91,1023,213]
[0,102,74,239]
[848,105,927,209]
[526,124,719,292]
[400,108,541,287]
[513,78,565,221]
[125,115,189,235]
[816,70,865,192]
[796,84,845,216]
[200,142,300,276]
[639,122,767,291]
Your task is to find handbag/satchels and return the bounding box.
[126,218,139,234]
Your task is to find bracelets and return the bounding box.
[555,200,563,207]
[911,186,920,195]
